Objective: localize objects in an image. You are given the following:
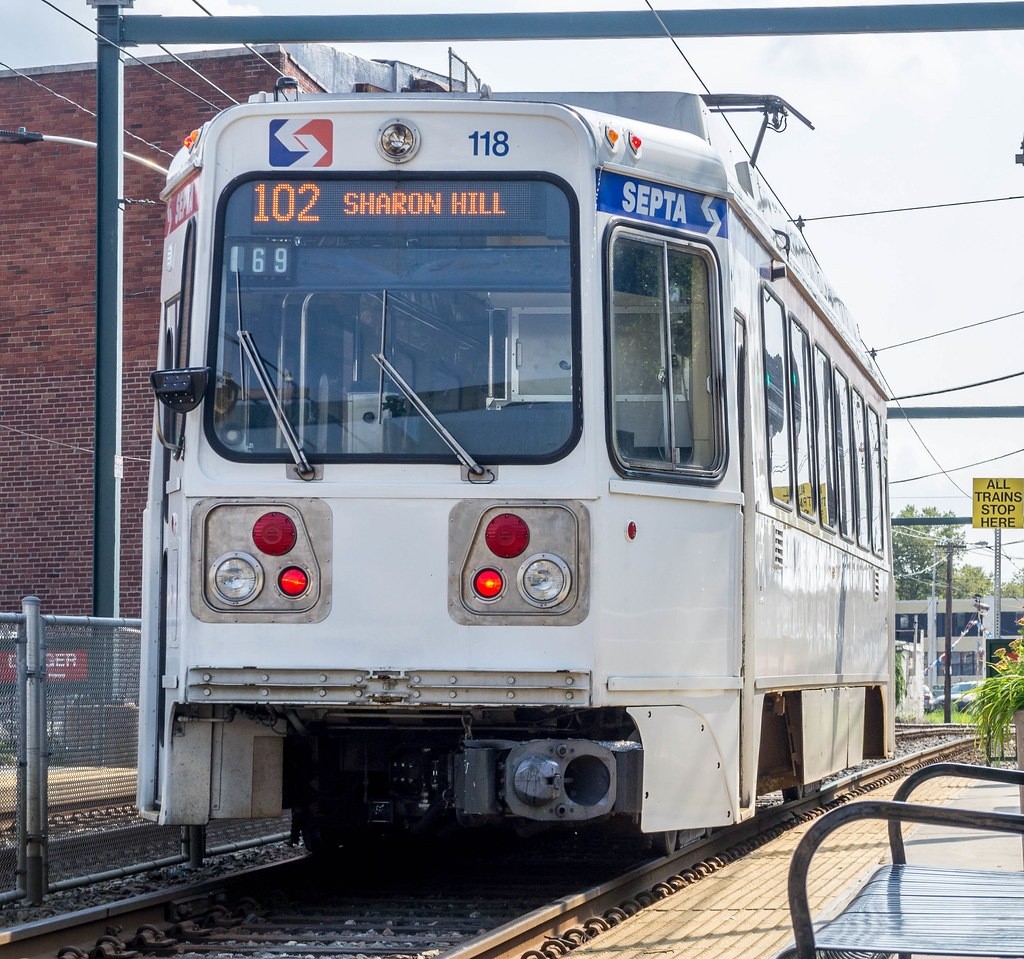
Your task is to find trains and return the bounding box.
[135,75,896,857]
